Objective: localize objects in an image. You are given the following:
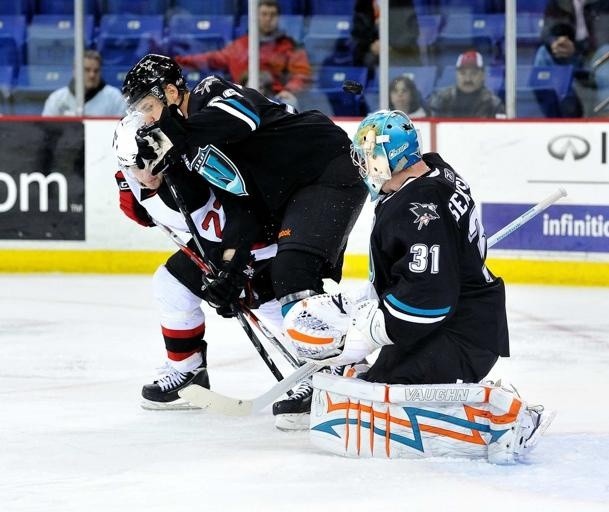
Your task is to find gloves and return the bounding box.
[136,119,177,176]
[199,261,259,318]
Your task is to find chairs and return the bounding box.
[0,1,609,119]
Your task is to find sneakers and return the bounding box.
[274,367,332,416]
[488,379,539,446]
[142,366,209,403]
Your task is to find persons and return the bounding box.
[174,0,315,110]
[113,110,333,416]
[350,0,421,79]
[42,50,128,118]
[532,0,609,117]
[432,48,506,117]
[389,74,428,117]
[284,108,553,466]
[122,53,371,380]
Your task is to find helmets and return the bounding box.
[350,109,423,202]
[112,111,153,167]
[121,54,189,113]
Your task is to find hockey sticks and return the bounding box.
[178,188,567,421]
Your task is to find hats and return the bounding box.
[455,51,485,69]
